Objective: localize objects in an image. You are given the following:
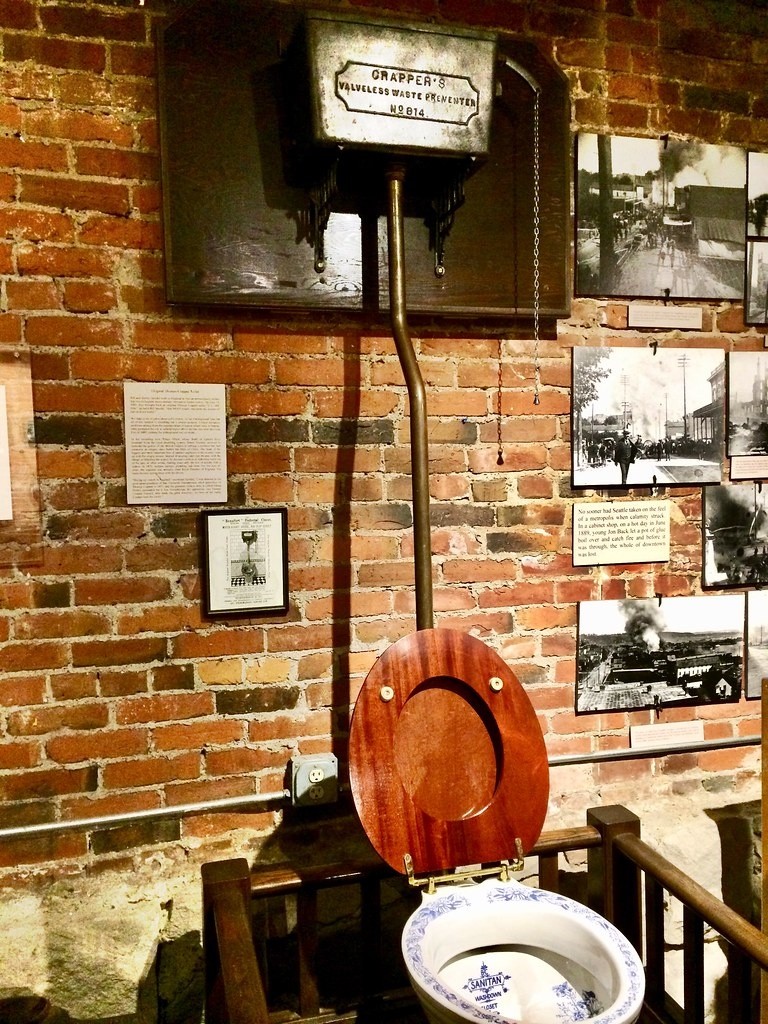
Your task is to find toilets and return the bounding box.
[345,624,645,1024]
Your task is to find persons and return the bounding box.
[580,425,715,487]
[614,206,677,269]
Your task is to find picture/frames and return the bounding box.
[747,243,768,329]
[571,124,749,304]
[742,143,768,242]
[742,588,767,705]
[567,345,726,489]
[572,589,746,715]
[196,504,293,623]
[726,349,768,458]
[729,455,768,484]
[700,482,767,592]
